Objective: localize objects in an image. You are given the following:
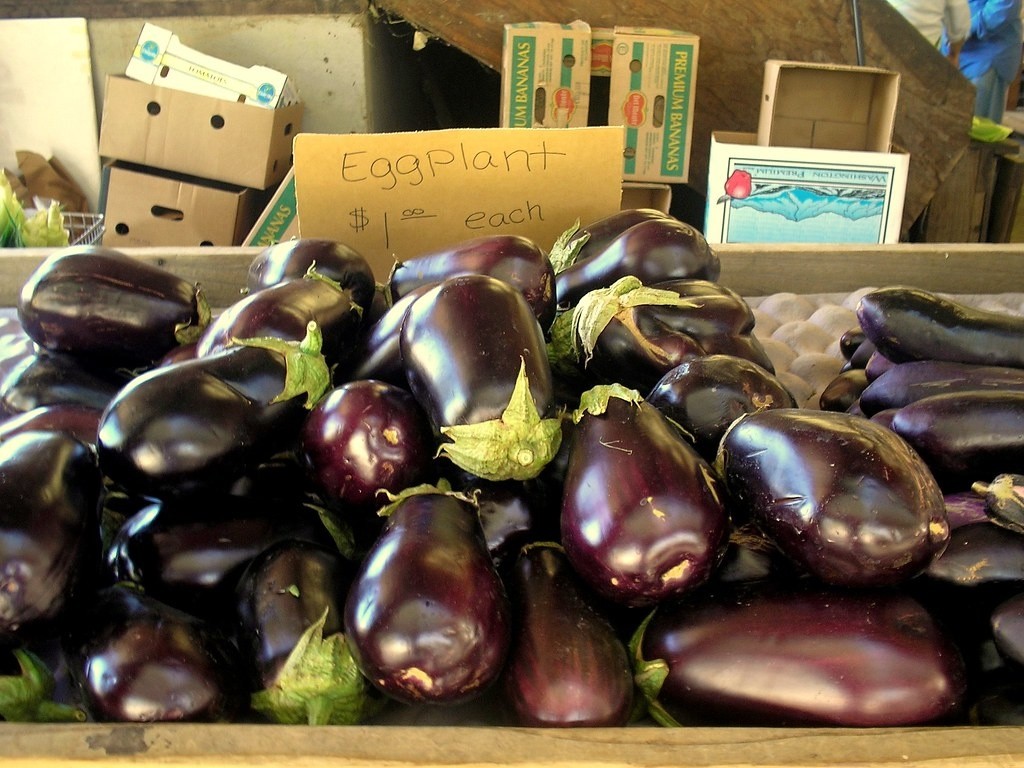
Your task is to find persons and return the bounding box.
[888,0,1024,125]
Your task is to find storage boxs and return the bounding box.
[499,19,701,213]
[99,73,305,190]
[704,59,911,244]
[98,158,248,247]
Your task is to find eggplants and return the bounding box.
[0,207,1024,724]
